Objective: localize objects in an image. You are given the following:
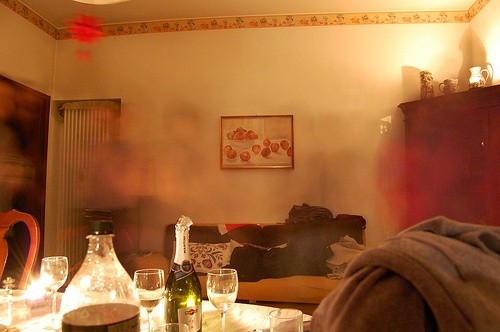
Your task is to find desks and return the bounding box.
[396,84,500,229]
[0,288,313,332]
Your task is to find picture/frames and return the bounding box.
[220,116,294,169]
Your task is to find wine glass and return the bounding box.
[133,268,165,332]
[40,256,68,321]
[165,215,203,332]
[206,268,238,332]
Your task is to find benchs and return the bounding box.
[162,221,365,315]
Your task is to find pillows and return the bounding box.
[168,241,232,274]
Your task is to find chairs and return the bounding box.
[0,209,41,290]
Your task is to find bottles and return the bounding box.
[59,220,149,332]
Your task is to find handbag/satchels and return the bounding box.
[221,245,265,282]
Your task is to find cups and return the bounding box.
[268,308,304,332]
[152,323,190,332]
[419,71,433,97]
[0,289,29,323]
[468,66,489,89]
[438,78,459,95]
